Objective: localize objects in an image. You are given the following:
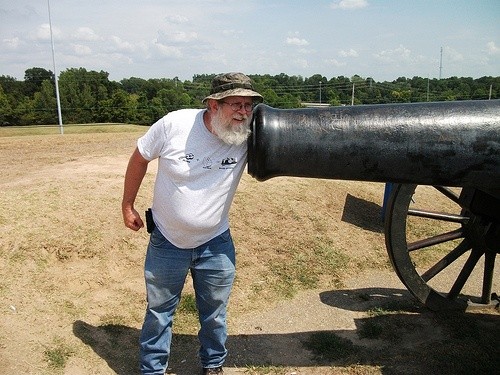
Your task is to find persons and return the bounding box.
[121,72,265,375]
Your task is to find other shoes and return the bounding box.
[203,366,223,375]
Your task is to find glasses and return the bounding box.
[219,101,253,112]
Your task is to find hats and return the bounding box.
[201,72,264,103]
